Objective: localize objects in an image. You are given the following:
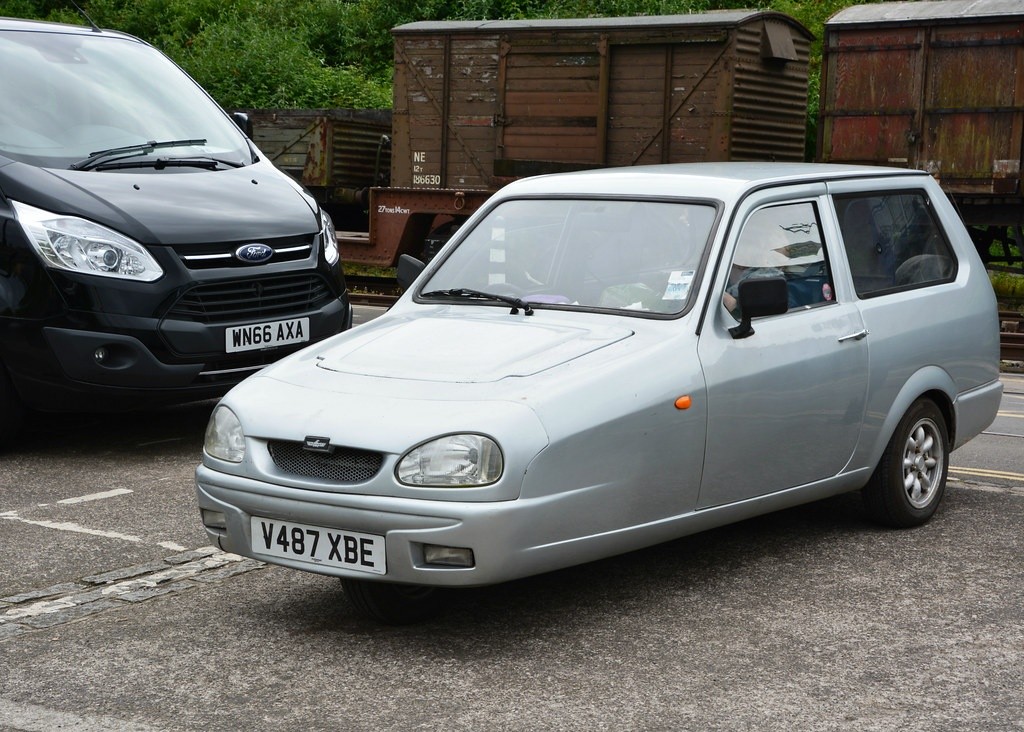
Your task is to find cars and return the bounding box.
[193,159,1004,630]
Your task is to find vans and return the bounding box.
[0,15,355,421]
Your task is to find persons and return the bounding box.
[723,227,788,318]
